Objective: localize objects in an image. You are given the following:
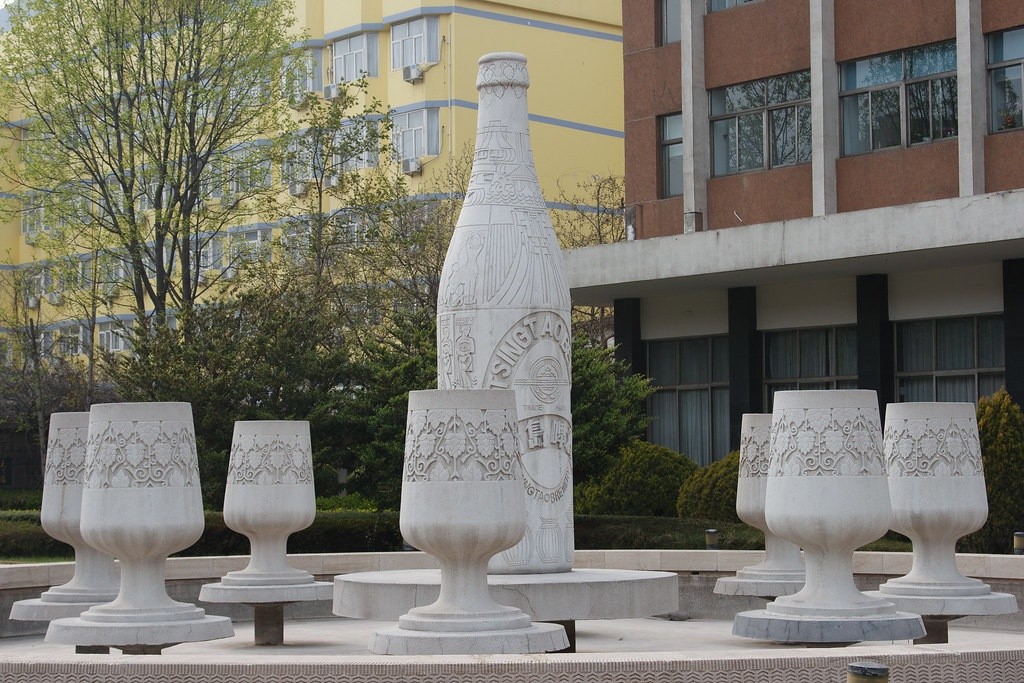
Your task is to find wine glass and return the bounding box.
[221,419,317,587]
[400,388,531,632]
[79,402,207,624]
[878,401,992,597]
[40,411,121,602]
[734,414,806,581]
[765,389,896,614]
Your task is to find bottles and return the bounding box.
[436,52,575,578]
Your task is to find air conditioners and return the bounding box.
[1,78,342,370]
[403,65,421,82]
[400,157,423,178]
